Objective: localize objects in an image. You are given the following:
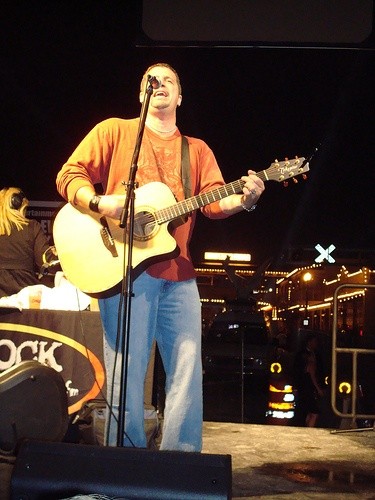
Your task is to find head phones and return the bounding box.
[10,192,25,210]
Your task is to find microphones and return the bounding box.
[147,74,161,89]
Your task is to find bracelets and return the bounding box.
[88,195,101,214]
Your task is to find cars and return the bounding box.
[205,313,273,384]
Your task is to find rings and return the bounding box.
[250,190,257,194]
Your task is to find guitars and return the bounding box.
[51,155,312,300]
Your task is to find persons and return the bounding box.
[56,63,265,451]
[292,334,325,427]
[0,186,63,299]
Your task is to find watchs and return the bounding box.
[241,195,258,212]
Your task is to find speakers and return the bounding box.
[10,438,233,500]
[0,358,69,455]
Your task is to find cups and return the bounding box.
[28,286,42,309]
